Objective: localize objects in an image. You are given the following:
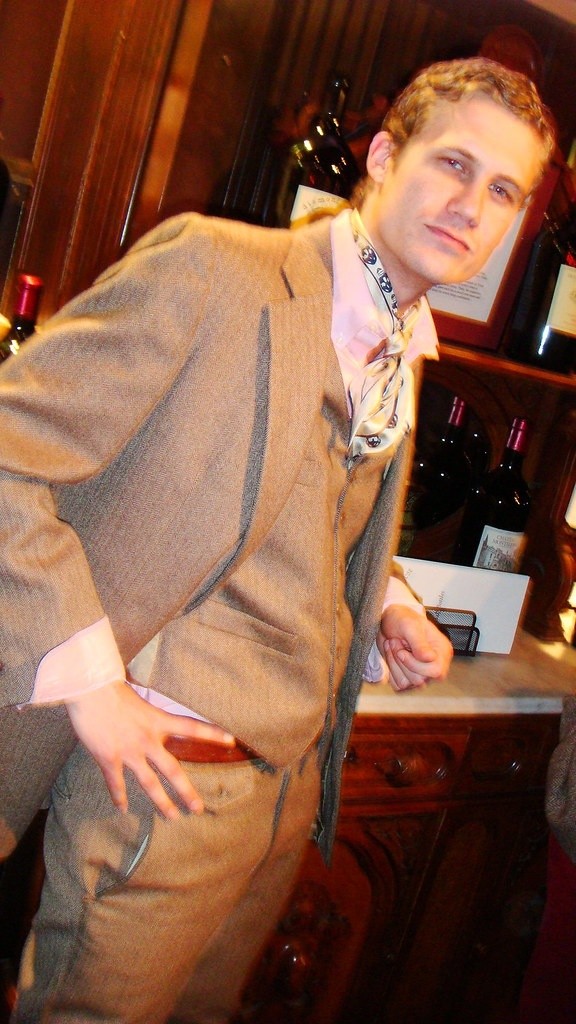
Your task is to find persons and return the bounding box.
[0,59,555,1023]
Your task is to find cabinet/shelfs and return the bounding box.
[0,0,575,1024]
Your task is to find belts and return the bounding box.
[163,734,263,764]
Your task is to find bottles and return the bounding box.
[450,415,533,572]
[498,201,576,375]
[404,394,473,528]
[262,71,358,222]
[0,272,46,366]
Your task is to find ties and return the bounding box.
[348,219,422,463]
